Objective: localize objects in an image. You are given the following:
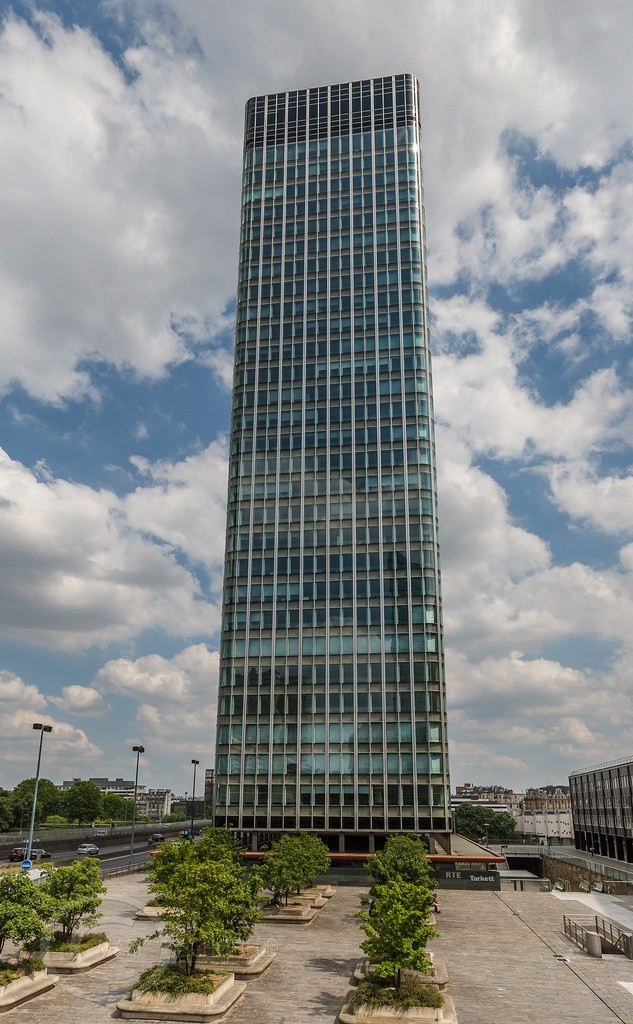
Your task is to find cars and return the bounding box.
[152,834,165,842]
[77,843,99,855]
[148,836,156,845]
[8,848,51,861]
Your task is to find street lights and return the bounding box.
[26,722,52,860]
[484,823,489,846]
[190,760,200,841]
[129,745,146,867]
